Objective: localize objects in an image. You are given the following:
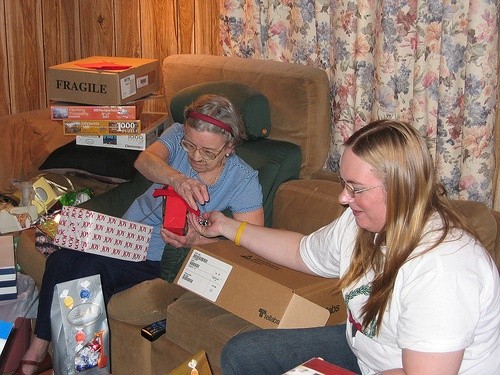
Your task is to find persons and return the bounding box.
[13,95,264,375]
[186,119,500,375]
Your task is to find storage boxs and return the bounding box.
[0,205,154,301]
[47,56,170,150]
[174,239,347,329]
[282,357,358,375]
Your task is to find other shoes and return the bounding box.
[15,353,54,375]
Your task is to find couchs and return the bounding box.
[0,55,330,375]
[107,180,500,375]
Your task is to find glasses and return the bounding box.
[180,132,229,160]
[340,178,382,198]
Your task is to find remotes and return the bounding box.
[141,319,167,342]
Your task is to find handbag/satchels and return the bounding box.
[51,273,112,375]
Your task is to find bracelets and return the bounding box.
[235,221,248,246]
